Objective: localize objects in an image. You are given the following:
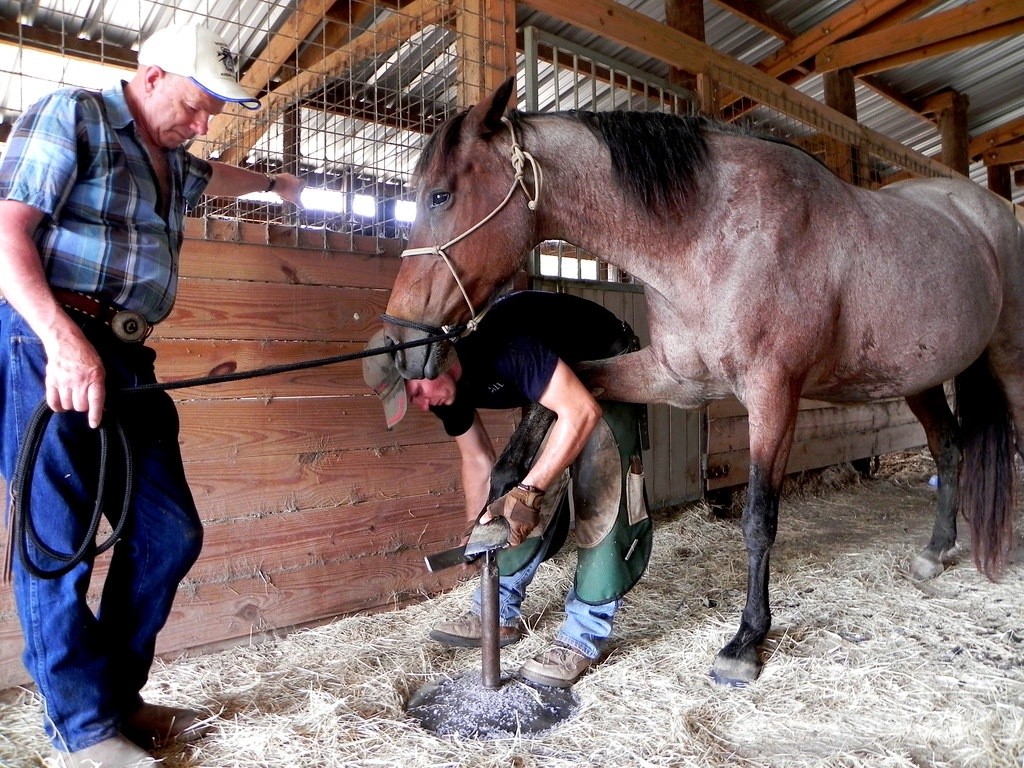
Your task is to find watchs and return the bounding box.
[263,172,277,192]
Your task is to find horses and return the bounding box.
[381,77,1024,687]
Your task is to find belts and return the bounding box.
[54,290,150,342]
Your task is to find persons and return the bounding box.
[362,289,653,688]
[0,23,307,768]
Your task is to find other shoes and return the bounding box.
[124,703,207,743]
[521,640,591,688]
[430,608,518,647]
[53,734,159,768]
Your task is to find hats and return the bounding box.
[138,24,261,110]
[362,328,408,428]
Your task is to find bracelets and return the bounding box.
[518,482,546,494]
